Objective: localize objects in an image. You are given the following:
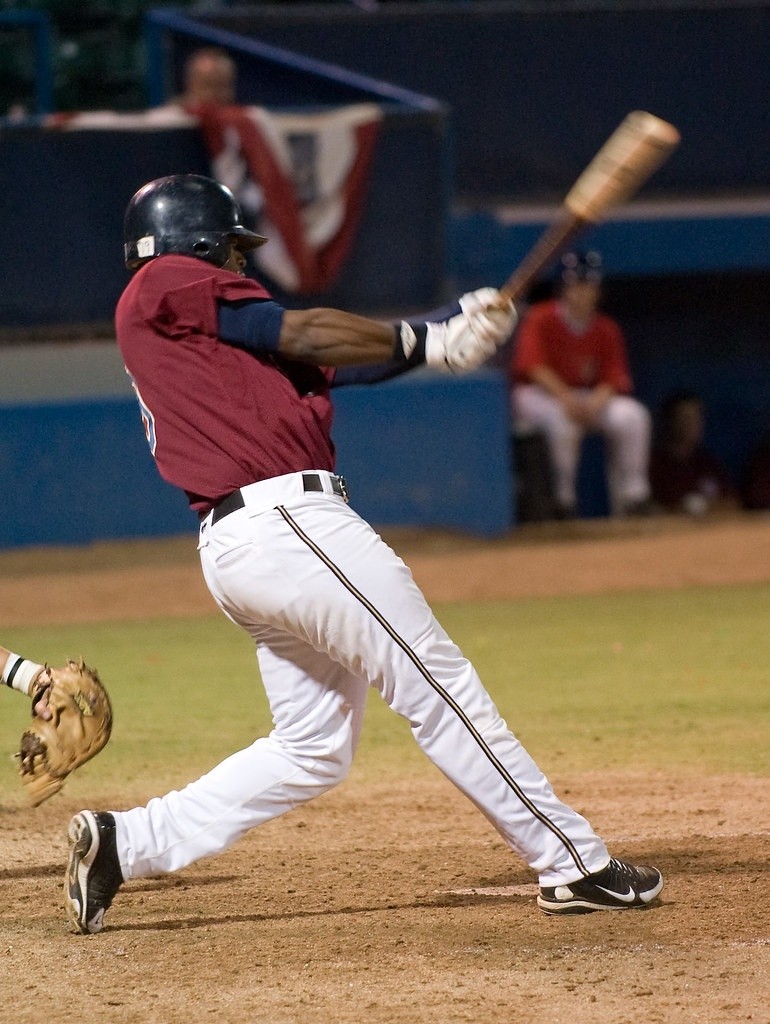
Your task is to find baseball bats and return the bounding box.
[448,114,677,373]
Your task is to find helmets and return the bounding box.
[122,172,269,268]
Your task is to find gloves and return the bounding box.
[393,288,524,374]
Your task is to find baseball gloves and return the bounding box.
[20,663,111,808]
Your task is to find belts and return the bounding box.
[197,474,352,531]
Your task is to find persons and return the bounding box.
[63,174,665,934]
[513,263,647,518]
[192,53,239,105]
[0,641,114,813]
[661,402,739,516]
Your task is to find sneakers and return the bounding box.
[63,806,122,937]
[535,854,667,915]
